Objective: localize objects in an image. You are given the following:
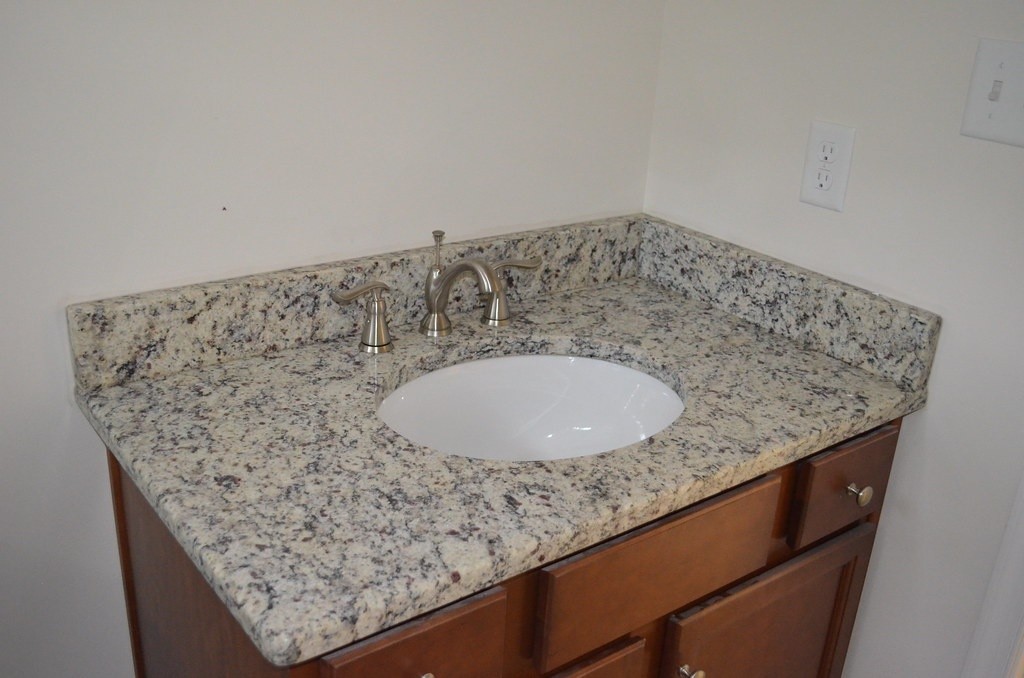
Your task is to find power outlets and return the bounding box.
[795,108,864,209]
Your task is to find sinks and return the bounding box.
[372,335,687,463]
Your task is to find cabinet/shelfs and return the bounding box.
[62,211,941,678]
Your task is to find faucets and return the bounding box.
[417,228,513,338]
